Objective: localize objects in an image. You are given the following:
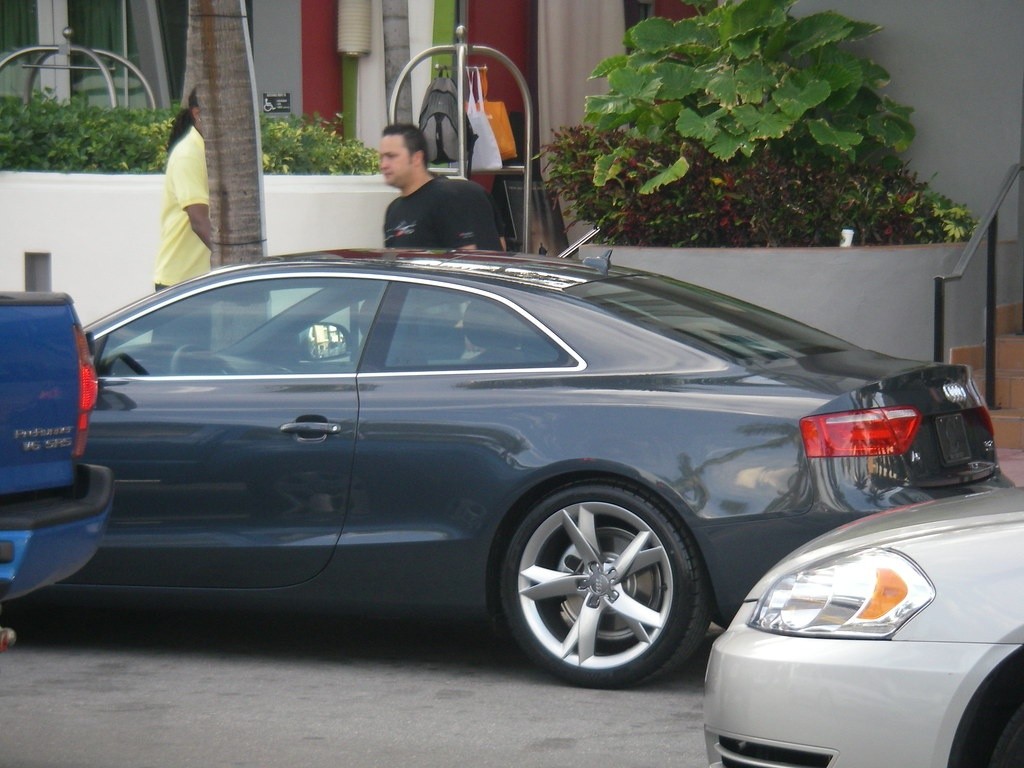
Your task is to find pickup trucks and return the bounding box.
[0,287,115,652]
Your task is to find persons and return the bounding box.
[154,87,211,292]
[378,121,506,254]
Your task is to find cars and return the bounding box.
[20,247,1018,690]
[700,485,1024,768]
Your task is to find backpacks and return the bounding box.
[418,63,479,165]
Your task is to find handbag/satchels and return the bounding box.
[464,65,519,161]
[464,65,503,173]
